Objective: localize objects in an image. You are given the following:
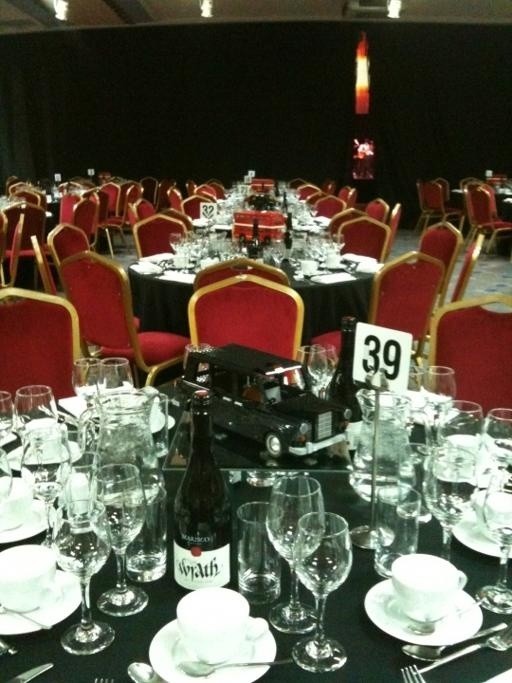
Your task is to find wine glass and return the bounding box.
[160,175,356,277]
[1,341,512,683]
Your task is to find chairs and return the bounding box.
[0,171,511,420]
